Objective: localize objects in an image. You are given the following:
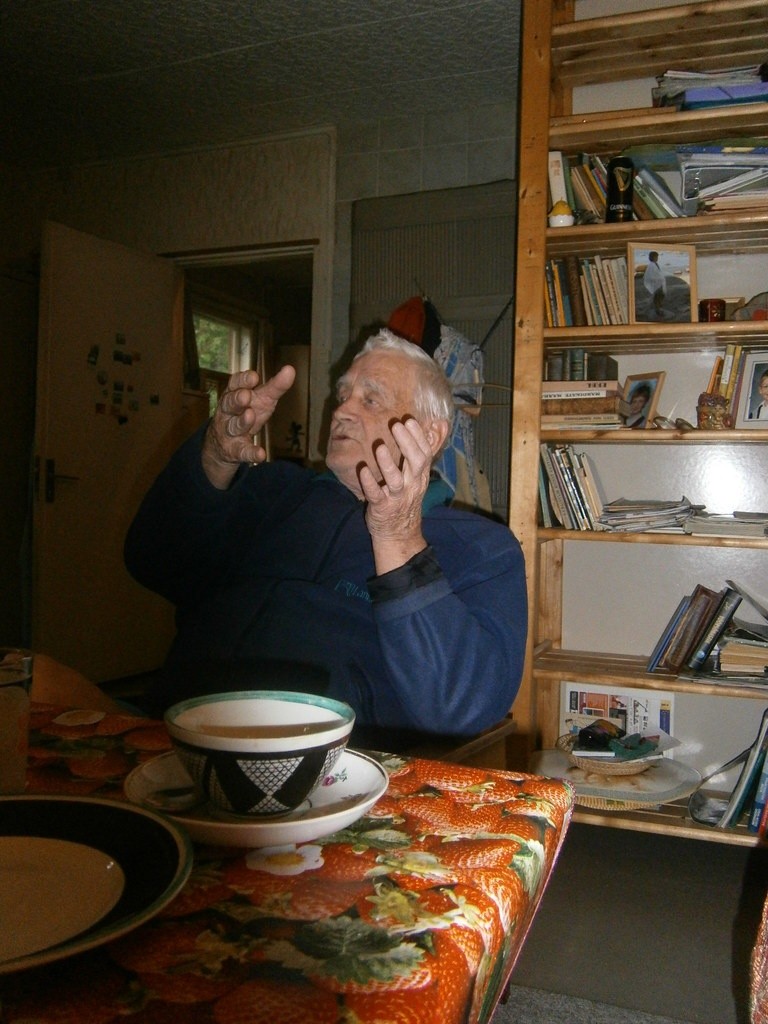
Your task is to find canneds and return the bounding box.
[605,155,633,223]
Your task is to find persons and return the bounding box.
[644,250,667,318]
[747,368,768,420]
[623,383,651,428]
[122,324,529,753]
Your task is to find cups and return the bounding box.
[0,647,34,794]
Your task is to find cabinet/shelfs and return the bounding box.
[504,1,768,853]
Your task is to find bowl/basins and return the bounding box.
[165,690,355,820]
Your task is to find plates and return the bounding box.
[0,793,193,975]
[123,747,389,846]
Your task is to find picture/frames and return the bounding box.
[619,370,667,429]
[626,241,698,323]
[733,351,768,430]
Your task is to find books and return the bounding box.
[539,341,633,433]
[714,703,768,833]
[644,576,767,689]
[570,738,616,758]
[649,63,768,111]
[549,128,768,231]
[544,241,628,329]
[701,341,750,432]
[533,442,768,538]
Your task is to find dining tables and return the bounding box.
[1,695,577,1024]
